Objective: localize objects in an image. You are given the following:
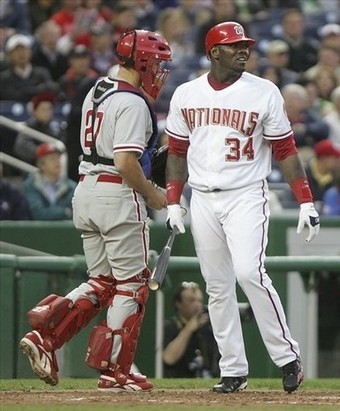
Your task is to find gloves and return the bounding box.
[296,200,320,241]
[166,203,186,235]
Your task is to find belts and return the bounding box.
[78,172,122,183]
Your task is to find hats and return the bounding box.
[313,139,340,159]
[35,142,67,159]
[6,34,33,51]
[318,21,340,38]
[69,43,91,60]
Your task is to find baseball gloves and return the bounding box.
[146,143,189,189]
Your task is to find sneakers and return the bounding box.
[212,373,249,393]
[97,372,154,393]
[19,329,59,386]
[283,354,305,393]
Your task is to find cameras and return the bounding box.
[198,303,251,336]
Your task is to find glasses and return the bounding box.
[181,281,199,289]
[278,48,288,55]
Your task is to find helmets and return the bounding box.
[204,21,256,58]
[115,26,172,100]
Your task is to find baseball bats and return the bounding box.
[147,207,187,291]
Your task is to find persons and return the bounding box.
[164,22,320,393]
[21,142,77,219]
[182,2,216,48]
[12,92,68,160]
[319,23,340,49]
[280,83,330,147]
[59,43,99,100]
[164,281,222,378]
[304,140,340,199]
[279,7,321,71]
[295,77,333,119]
[134,0,158,31]
[152,6,189,66]
[303,47,340,82]
[19,28,172,394]
[1,33,63,102]
[110,6,137,42]
[254,39,291,88]
[303,65,337,100]
[31,19,70,80]
[323,87,340,149]
[0,162,33,220]
[197,1,252,57]
[51,0,113,48]
